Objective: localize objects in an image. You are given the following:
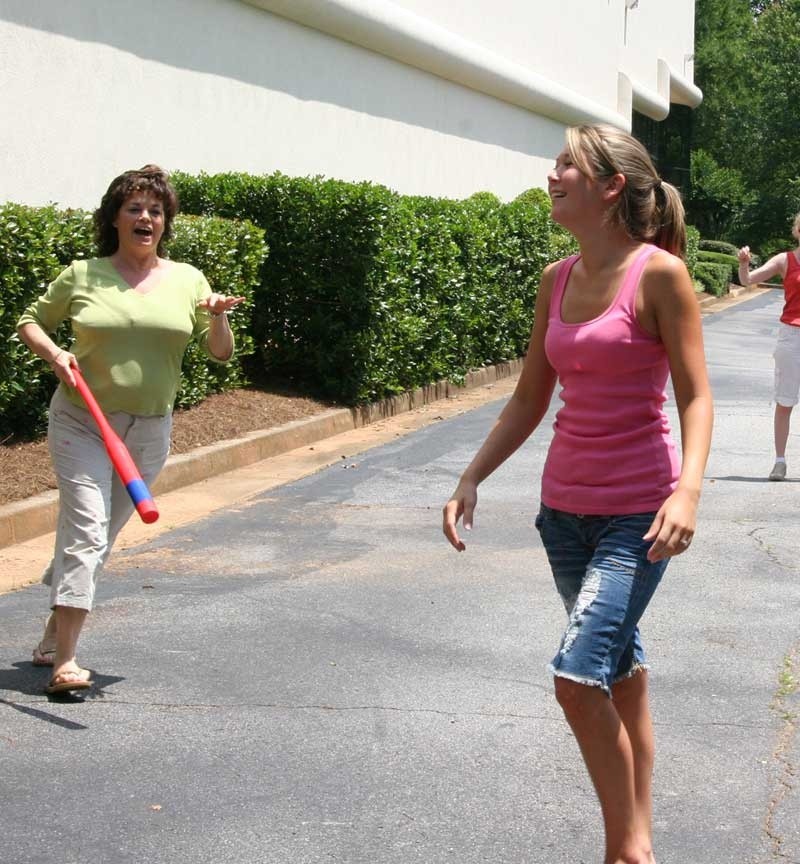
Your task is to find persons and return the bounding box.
[737,213,800,481]
[16,164,246,693]
[440,123,715,864]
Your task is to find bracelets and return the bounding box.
[49,350,64,365]
[208,312,225,320]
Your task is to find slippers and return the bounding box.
[46,668,91,692]
[32,641,57,664]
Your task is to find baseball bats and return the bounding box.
[68,363,159,525]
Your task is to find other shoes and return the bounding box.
[769,462,786,480]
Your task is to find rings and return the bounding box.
[680,539,687,544]
